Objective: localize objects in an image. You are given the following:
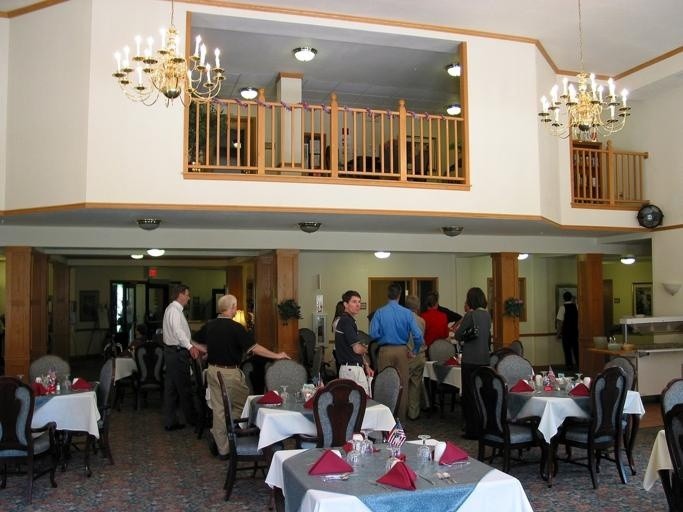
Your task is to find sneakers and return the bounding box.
[204,430,228,460]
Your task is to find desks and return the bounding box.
[638,429,676,509]
[242,388,398,449]
[496,375,613,481]
[26,389,101,477]
[263,436,538,512]
[421,357,465,429]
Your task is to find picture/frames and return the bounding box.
[555,283,578,329]
[405,135,436,172]
[632,282,652,316]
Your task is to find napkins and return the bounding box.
[374,460,419,491]
[71,376,93,390]
[29,382,48,396]
[439,439,468,466]
[256,390,286,405]
[304,392,318,409]
[308,449,355,475]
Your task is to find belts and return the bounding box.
[342,362,365,368]
[210,362,240,369]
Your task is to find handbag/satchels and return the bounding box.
[460,326,480,340]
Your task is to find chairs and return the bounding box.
[535,365,630,489]
[93,331,252,412]
[312,378,368,448]
[600,353,637,472]
[29,353,71,380]
[508,338,526,357]
[426,337,456,362]
[567,382,592,397]
[59,356,118,476]
[471,366,553,486]
[490,352,549,472]
[189,350,210,439]
[658,377,681,511]
[370,365,403,418]
[214,371,271,501]
[266,360,309,391]
[509,378,535,394]
[2,374,61,504]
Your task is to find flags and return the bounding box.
[386,420,407,447]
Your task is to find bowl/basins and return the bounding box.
[621,344,635,350]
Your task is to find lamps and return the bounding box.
[293,47,317,62]
[240,87,258,100]
[442,226,463,236]
[298,222,321,232]
[113,0,225,108]
[446,104,462,115]
[620,257,635,265]
[538,0,632,142]
[137,219,160,230]
[663,283,681,296]
[447,63,461,76]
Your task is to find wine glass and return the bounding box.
[279,381,308,405]
[15,373,71,395]
[344,427,430,475]
[526,370,582,393]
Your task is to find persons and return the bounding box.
[161,285,212,431]
[335,290,374,398]
[404,286,491,430]
[368,283,423,430]
[191,295,292,461]
[556,292,577,369]
[332,302,344,370]
